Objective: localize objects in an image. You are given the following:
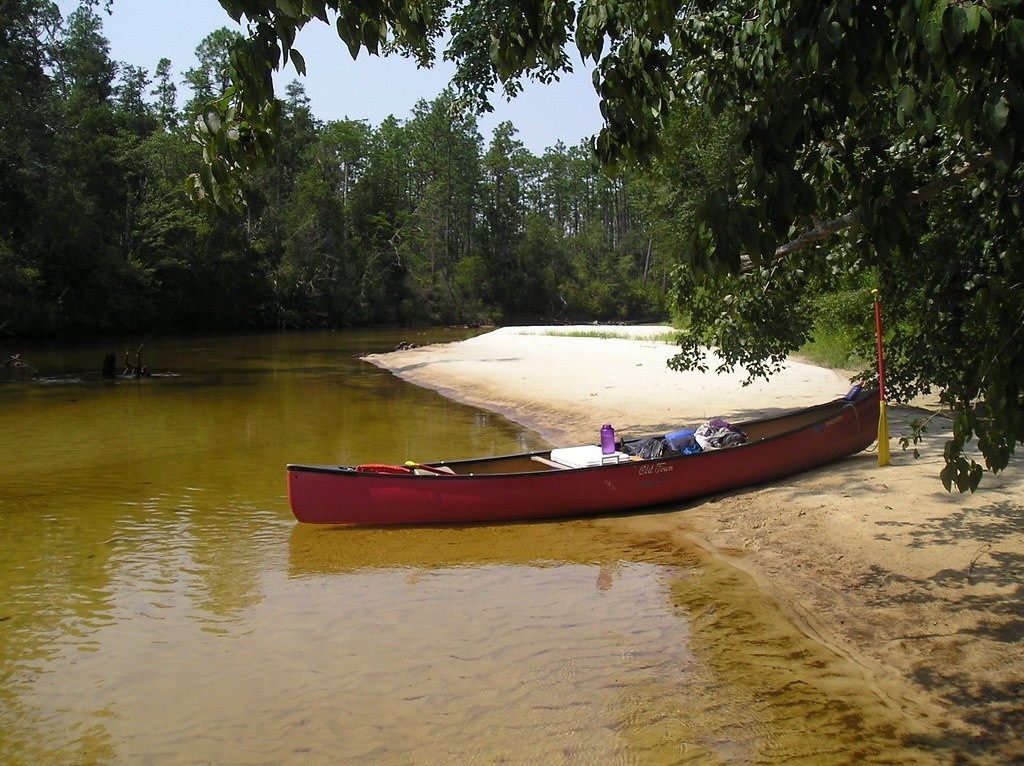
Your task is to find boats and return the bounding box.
[280,381,885,529]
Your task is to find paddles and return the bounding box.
[871,289,891,467]
[405,461,456,476]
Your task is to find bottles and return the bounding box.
[845,382,864,400]
[601,425,615,455]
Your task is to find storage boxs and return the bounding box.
[665,428,696,451]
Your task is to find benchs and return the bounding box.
[550,444,630,468]
